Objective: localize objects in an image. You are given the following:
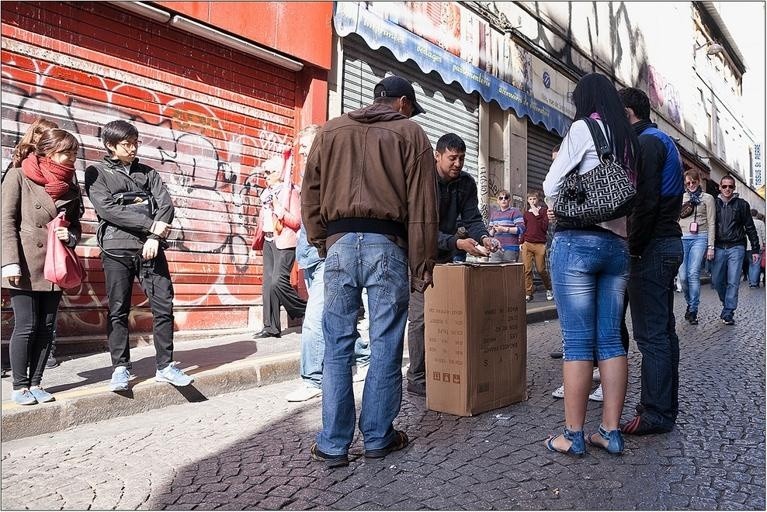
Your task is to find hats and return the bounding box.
[374,76,425,118]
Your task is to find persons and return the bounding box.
[285,123,373,403]
[545,72,639,455]
[519,191,554,302]
[0,127,81,406]
[0,117,84,370]
[406,132,504,395]
[306,77,441,468]
[678,167,765,324]
[252,155,306,339]
[85,120,194,393]
[617,86,685,437]
[488,191,524,264]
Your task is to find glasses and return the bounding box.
[117,141,142,147]
[499,197,509,200]
[722,185,734,189]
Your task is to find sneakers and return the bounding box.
[526,289,734,460]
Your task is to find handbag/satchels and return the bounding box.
[43,212,84,288]
[553,155,634,231]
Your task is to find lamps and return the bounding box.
[696,41,724,56]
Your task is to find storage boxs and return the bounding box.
[423,263,527,416]
